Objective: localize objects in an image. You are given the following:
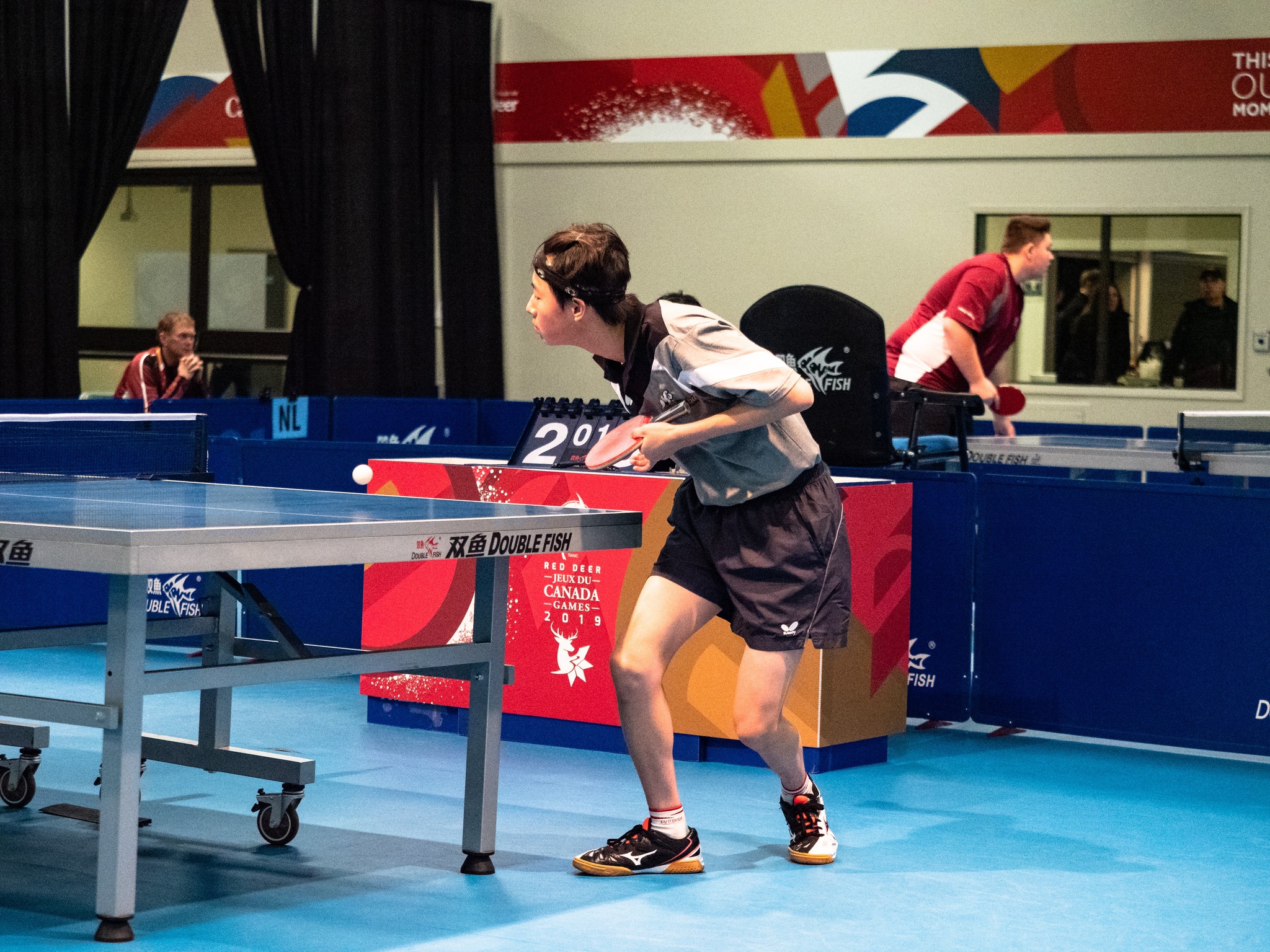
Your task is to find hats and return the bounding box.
[1199,266,1227,282]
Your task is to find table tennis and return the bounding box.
[353,464,374,486]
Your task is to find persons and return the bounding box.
[657,290,701,306]
[1168,270,1238,390]
[886,216,1055,437]
[1056,270,1131,384]
[113,312,205,414]
[525,223,852,877]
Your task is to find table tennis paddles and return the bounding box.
[988,384,1026,417]
[584,400,692,471]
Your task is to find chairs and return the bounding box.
[740,284,986,471]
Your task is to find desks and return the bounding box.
[949,410,1270,488]
[0,413,641,945]
[361,458,912,773]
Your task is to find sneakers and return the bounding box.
[572,815,705,875]
[779,773,838,864]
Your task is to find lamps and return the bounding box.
[120,186,139,222]
